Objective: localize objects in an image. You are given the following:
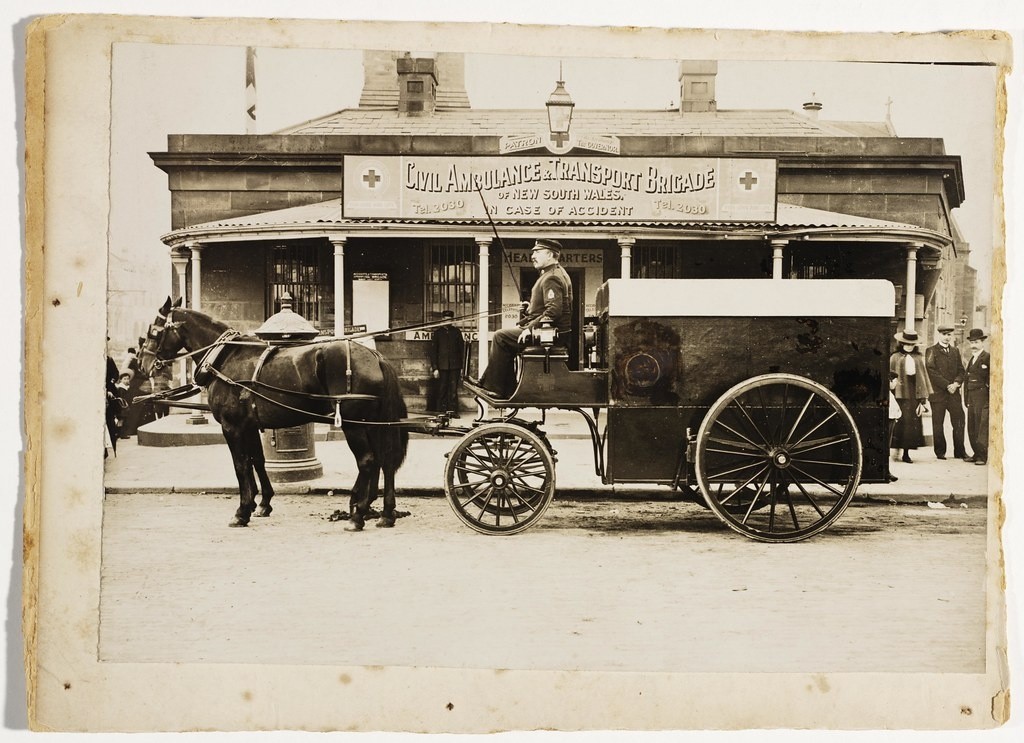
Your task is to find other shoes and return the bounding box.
[889,472,898,482]
[450,412,460,418]
[964,456,977,462]
[902,456,913,463]
[937,456,946,460]
[975,460,986,465]
[955,454,970,459]
[468,376,482,388]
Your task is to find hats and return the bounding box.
[938,325,954,333]
[531,236,563,253]
[894,330,920,344]
[967,329,987,340]
[442,310,454,317]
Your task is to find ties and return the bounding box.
[945,348,948,354]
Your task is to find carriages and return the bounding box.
[136,279,897,543]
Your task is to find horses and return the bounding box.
[138,296,409,532]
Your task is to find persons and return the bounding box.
[466,237,573,401]
[964,329,990,465]
[429,310,465,420]
[925,325,971,460]
[890,329,935,463]
[105,335,173,440]
